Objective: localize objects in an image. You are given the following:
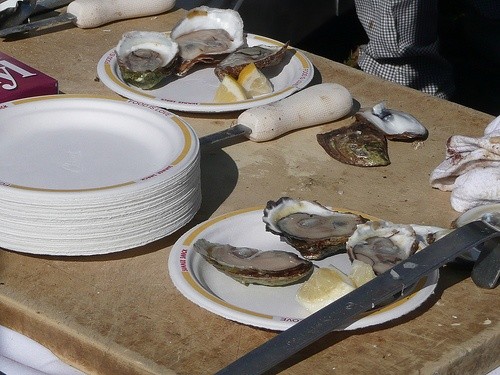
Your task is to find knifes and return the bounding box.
[199,81,354,147]
[0,0,177,39]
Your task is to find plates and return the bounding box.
[96,32,316,114]
[0,93,203,257]
[168,203,440,332]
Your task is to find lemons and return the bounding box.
[212,74,247,102]
[237,63,274,97]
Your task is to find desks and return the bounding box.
[0,0,500,374]
